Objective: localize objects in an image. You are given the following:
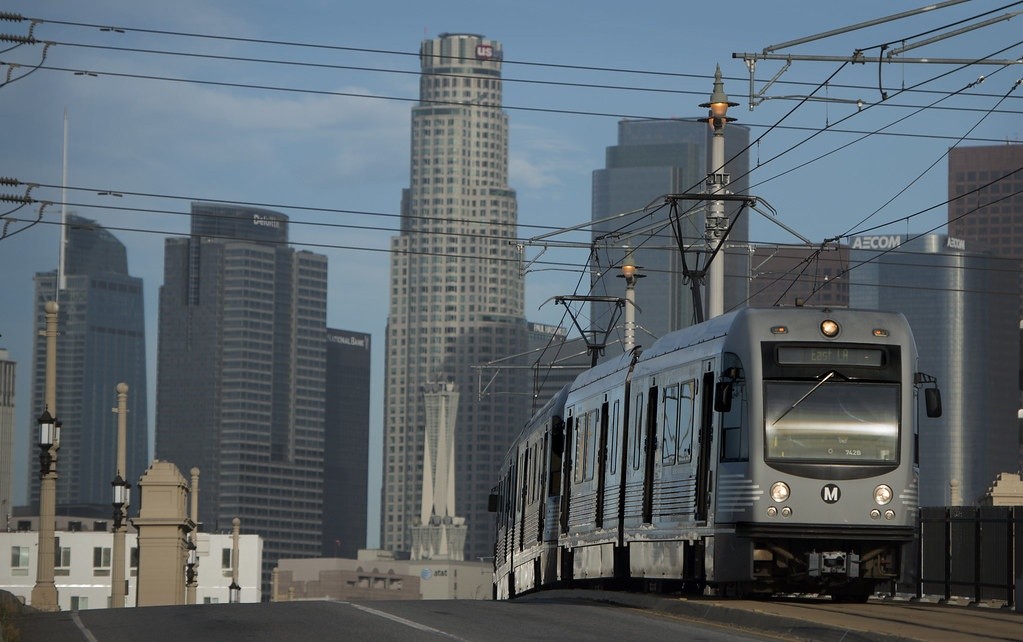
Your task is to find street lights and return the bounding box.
[183,466,200,608]
[229,518,241,604]
[31,298,62,614]
[106,381,129,610]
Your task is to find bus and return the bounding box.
[485,306,944,604]
